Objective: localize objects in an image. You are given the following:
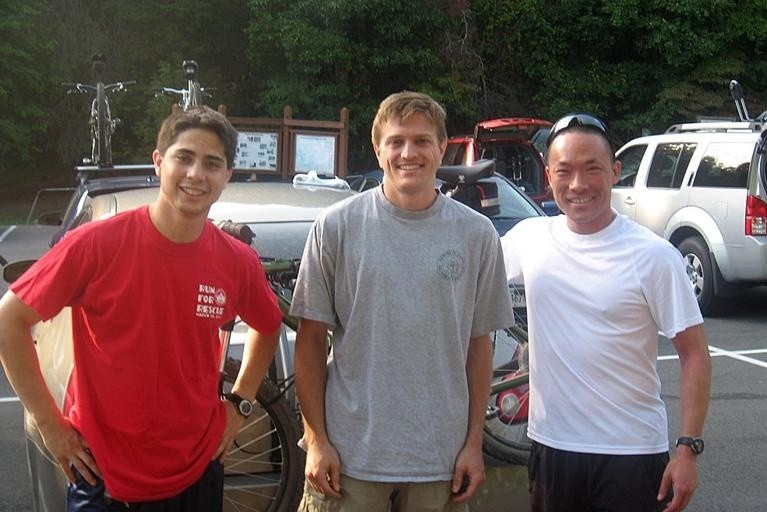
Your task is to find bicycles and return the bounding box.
[217,158,532,512]
[62,53,218,171]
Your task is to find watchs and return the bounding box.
[222,390,254,420]
[675,435,705,454]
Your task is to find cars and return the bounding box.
[0,174,362,512]
[346,169,560,379]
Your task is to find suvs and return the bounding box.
[439,118,554,215]
[611,118,767,317]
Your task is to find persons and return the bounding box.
[0,104,284,511]
[287,88,519,512]
[502,111,714,511]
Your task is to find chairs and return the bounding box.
[647,156,673,186]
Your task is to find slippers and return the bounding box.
[545,114,612,150]
